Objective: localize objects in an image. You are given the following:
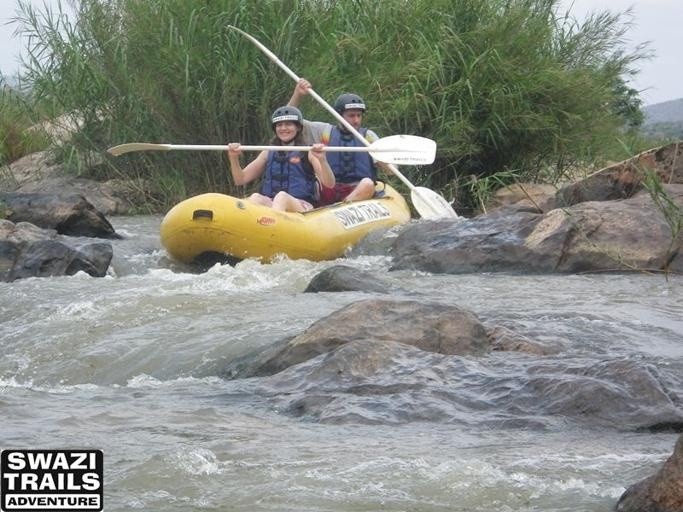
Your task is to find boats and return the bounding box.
[159,181,411,273]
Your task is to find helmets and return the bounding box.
[334,94,366,115]
[272,106,304,132]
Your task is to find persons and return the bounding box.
[286,77,389,202]
[226,105,336,212]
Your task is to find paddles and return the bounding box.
[107,135,436,166]
[227,25,457,219]
